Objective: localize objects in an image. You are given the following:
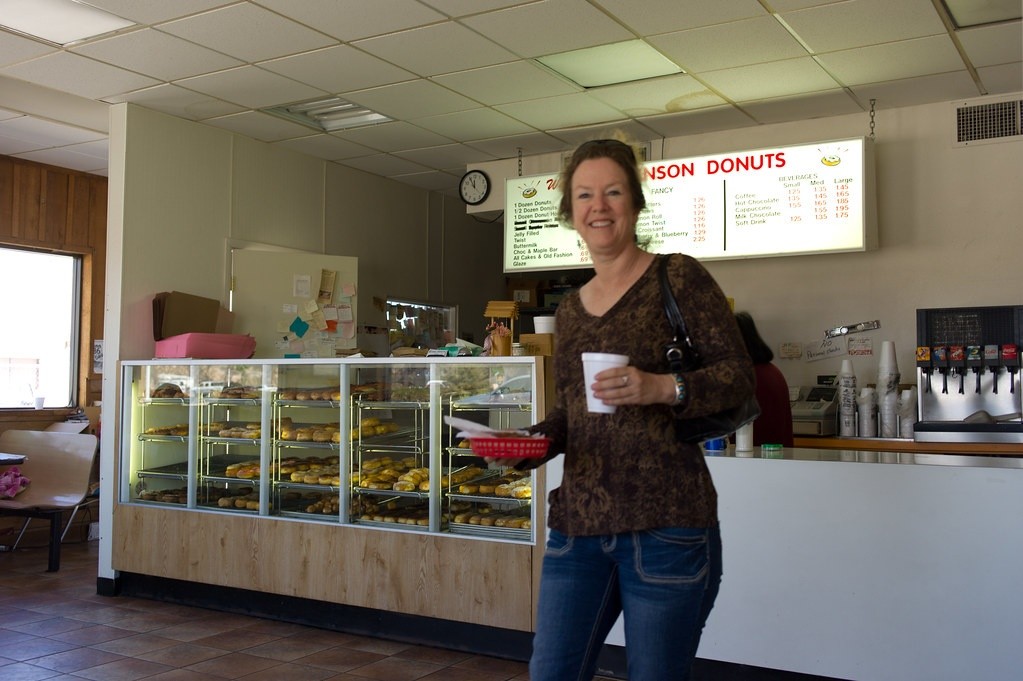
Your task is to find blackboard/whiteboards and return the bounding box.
[231,249,359,359]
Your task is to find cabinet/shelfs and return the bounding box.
[111,353,560,661]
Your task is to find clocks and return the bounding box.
[459,170,490,205]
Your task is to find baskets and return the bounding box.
[467,437,554,458]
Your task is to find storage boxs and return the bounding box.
[518,333,554,357]
[509,276,573,308]
[154,332,256,359]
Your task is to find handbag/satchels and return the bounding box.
[657,252,761,447]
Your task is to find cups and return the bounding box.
[533,316,555,334]
[582,351,629,414]
[736,422,753,451]
[840,341,917,438]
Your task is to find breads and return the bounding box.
[139,381,532,531]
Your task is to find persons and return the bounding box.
[485,139,760,680]
[734,310,794,448]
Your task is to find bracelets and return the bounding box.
[670,372,686,406]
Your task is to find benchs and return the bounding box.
[0,430,98,573]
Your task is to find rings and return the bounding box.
[623,376,628,383]
